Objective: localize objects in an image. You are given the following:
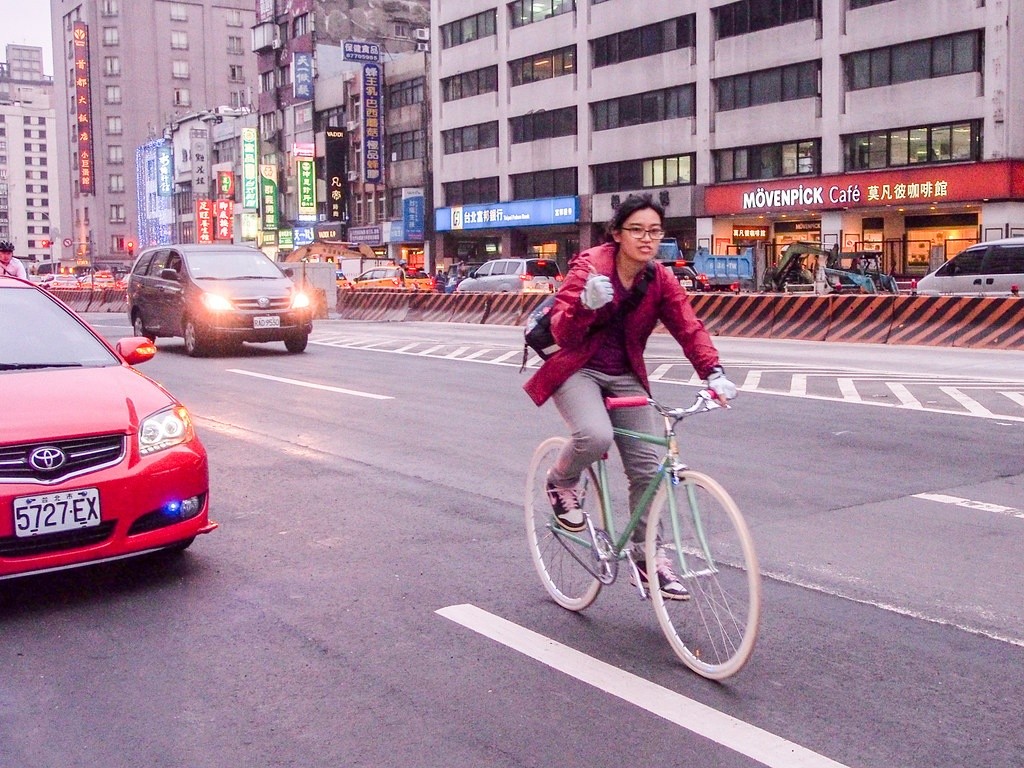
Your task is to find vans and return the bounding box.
[455,258,562,293]
[916,239,1024,295]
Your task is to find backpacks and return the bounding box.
[520,291,562,374]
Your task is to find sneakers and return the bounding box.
[545,478,588,532]
[629,558,690,601]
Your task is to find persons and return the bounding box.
[522,194,738,601]
[0,239,26,280]
[396,259,448,291]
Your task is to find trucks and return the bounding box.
[656,238,754,294]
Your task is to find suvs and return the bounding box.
[126,244,313,357]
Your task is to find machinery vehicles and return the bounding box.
[758,242,898,294]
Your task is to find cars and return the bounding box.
[82,275,114,290]
[349,267,434,292]
[1,276,220,582]
[38,274,79,288]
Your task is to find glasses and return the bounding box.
[620,223,665,240]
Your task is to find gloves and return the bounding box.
[708,371,738,400]
[577,270,613,309]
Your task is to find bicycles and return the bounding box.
[525,390,758,681]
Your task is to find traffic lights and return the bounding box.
[128,242,134,256]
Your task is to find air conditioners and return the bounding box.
[347,171,357,181]
[262,131,272,141]
[272,39,282,50]
[347,121,357,132]
[415,28,429,41]
[343,71,354,83]
[417,42,429,51]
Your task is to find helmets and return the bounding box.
[0,240,15,252]
